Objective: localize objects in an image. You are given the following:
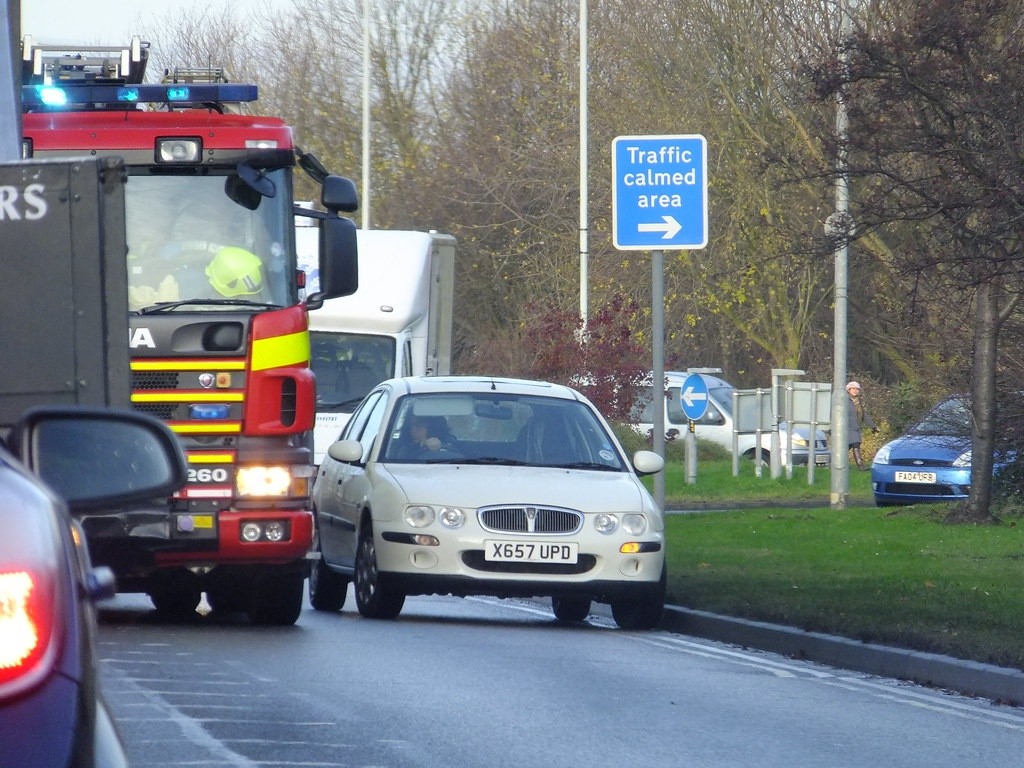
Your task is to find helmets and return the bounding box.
[204,247,265,297]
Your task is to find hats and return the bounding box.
[845,380,861,391]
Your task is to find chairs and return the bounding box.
[346,369,373,397]
[518,422,561,462]
[313,367,339,396]
[396,406,449,453]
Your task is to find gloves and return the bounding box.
[870,428,879,435]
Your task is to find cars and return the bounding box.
[302,375,670,627]
[869,389,1024,509]
[0,407,189,768]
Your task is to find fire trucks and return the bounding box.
[0,0,365,622]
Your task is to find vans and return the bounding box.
[564,366,834,470]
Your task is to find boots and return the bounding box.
[853,448,870,471]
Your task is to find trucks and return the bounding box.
[268,198,460,580]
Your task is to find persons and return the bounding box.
[845,381,879,472]
[397,407,450,460]
[336,341,388,395]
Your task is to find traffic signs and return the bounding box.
[611,134,711,252]
